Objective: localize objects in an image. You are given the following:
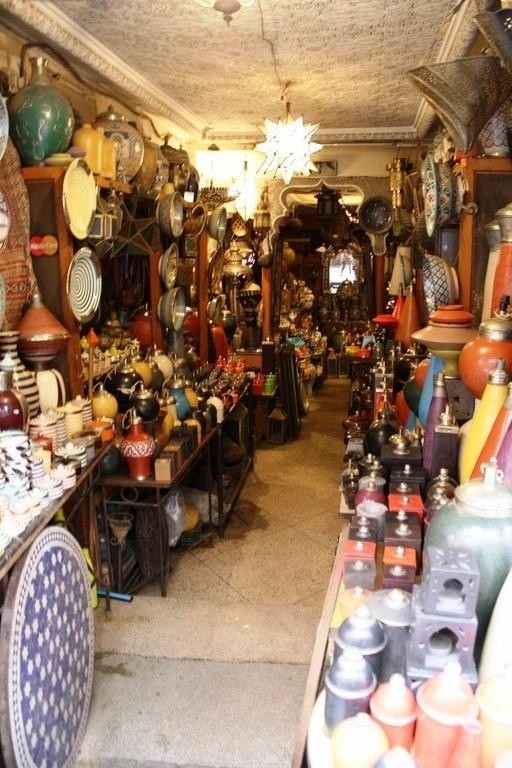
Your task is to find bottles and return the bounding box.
[424,357,511,491]
[321,587,486,767]
[72,122,117,178]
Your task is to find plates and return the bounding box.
[60,156,104,325]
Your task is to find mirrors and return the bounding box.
[321,237,365,296]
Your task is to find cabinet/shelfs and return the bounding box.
[22,166,207,400]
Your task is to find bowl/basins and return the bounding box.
[421,253,461,313]
[155,191,187,332]
[207,206,227,322]
[359,197,395,235]
[422,154,466,238]
